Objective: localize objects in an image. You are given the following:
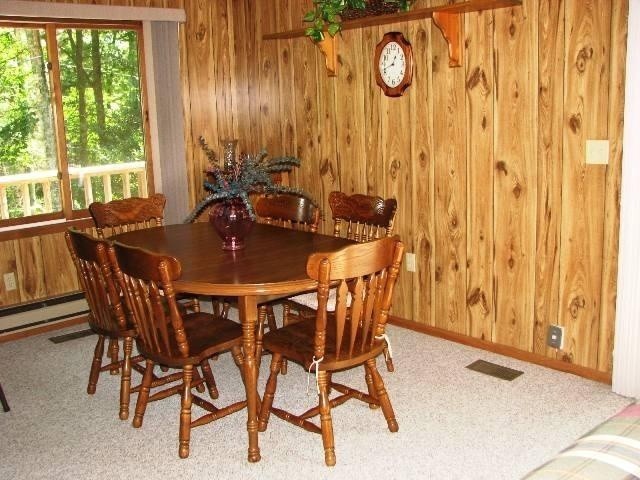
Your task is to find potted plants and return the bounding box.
[301,0,410,42]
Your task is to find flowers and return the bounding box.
[184,137,318,227]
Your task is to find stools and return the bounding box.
[88,192,200,315]
[252,191,321,363]
[279,192,400,373]
[65,226,148,420]
[101,236,263,460]
[259,235,404,468]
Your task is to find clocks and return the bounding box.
[373,32,414,98]
